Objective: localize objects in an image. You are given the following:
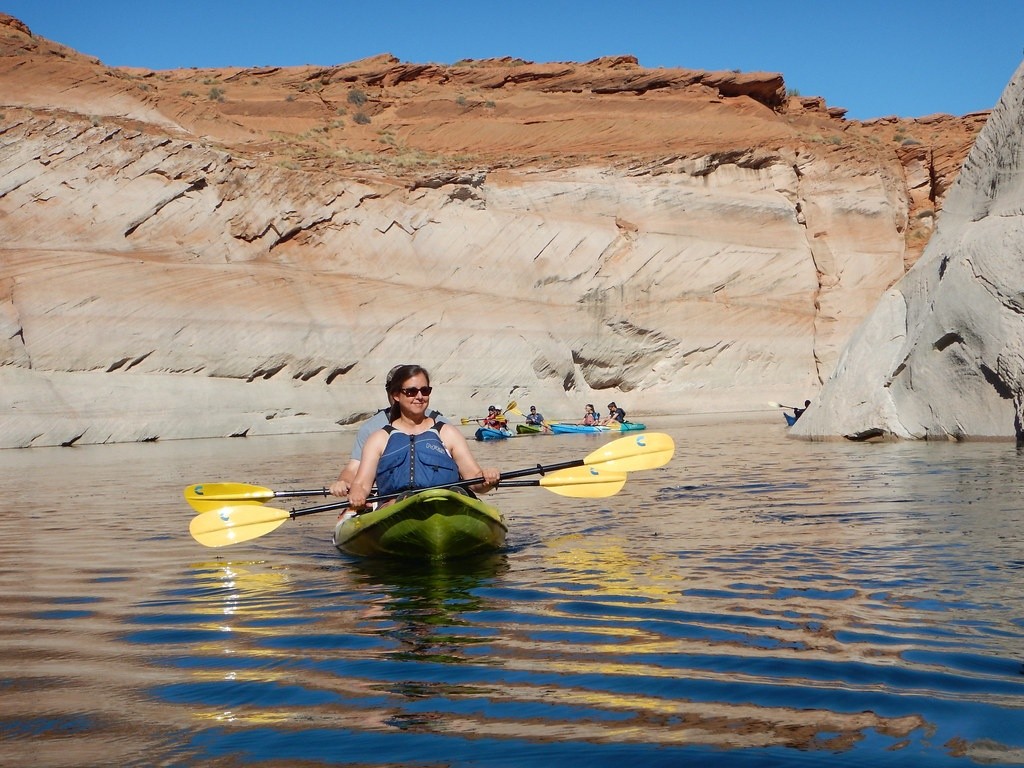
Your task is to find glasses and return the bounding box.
[400,386,433,397]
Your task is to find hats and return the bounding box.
[386,365,403,387]
[488,406,495,410]
[495,405,502,410]
[530,406,535,410]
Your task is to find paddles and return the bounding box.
[182,464,628,514]
[460,414,505,425]
[502,400,517,415]
[188,431,675,548]
[545,420,621,431]
[767,401,805,411]
[510,408,552,431]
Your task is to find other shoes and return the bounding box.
[394,490,415,503]
[449,486,470,497]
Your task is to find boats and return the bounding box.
[475,423,515,442]
[548,420,646,433]
[782,411,797,427]
[514,419,560,434]
[329,485,512,562]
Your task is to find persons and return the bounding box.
[793,400,811,419]
[476,405,508,432]
[601,402,625,427]
[526,405,548,429]
[575,404,599,426]
[328,364,500,518]
